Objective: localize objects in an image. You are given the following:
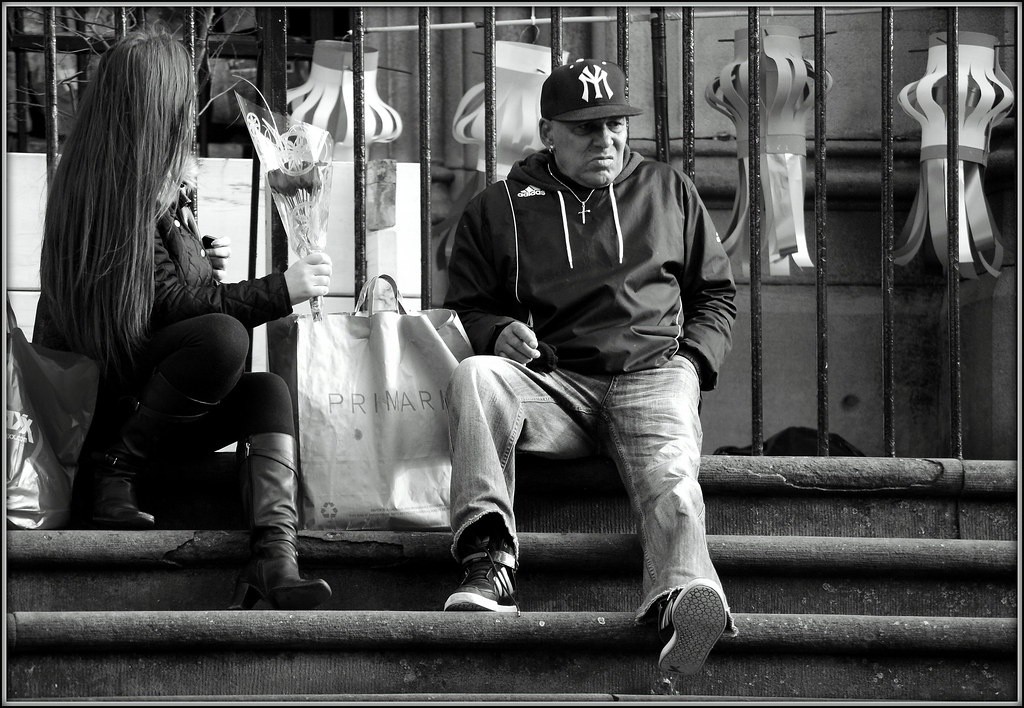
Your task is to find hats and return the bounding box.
[541,58,644,123]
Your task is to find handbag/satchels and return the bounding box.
[8,294,100,531]
[267,274,475,533]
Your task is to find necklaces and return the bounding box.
[548,163,597,224]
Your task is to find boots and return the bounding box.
[71,389,165,527]
[227,433,335,609]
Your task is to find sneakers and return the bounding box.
[657,576,728,677]
[442,535,520,613]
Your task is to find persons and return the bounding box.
[444,56,740,677]
[25,25,335,609]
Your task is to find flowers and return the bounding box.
[261,128,330,324]
